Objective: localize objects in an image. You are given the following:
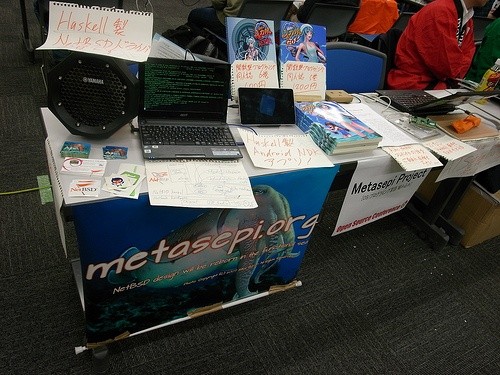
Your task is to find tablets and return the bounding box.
[238,88,296,126]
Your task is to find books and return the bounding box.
[224,16,279,104]
[280,20,326,101]
[294,101,384,156]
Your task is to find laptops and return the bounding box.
[137,56,242,159]
[376,89,500,116]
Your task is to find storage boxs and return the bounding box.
[417,167,500,249]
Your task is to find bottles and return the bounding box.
[473,58,500,105]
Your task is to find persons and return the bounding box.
[459,5,500,90]
[291,0,360,42]
[387,0,488,91]
[187,0,244,39]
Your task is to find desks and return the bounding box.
[40,90,500,375]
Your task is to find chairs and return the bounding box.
[186,0,496,94]
